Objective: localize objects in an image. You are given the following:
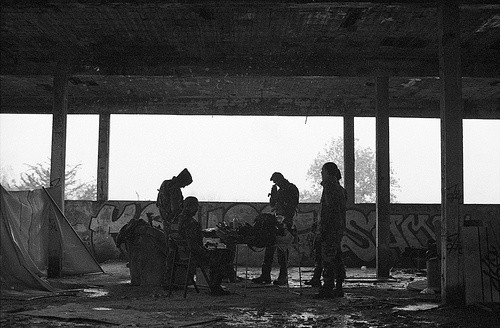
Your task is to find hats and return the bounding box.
[184,196,201,208]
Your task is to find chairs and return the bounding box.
[168,223,216,299]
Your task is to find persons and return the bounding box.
[157,168,193,242]
[252,172,300,285]
[305,162,347,298]
[171,196,231,296]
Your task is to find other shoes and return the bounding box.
[209,284,230,296]
[251,274,272,284]
[273,275,288,284]
[304,277,322,287]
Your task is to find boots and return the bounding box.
[319,276,345,298]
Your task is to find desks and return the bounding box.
[232,241,304,298]
